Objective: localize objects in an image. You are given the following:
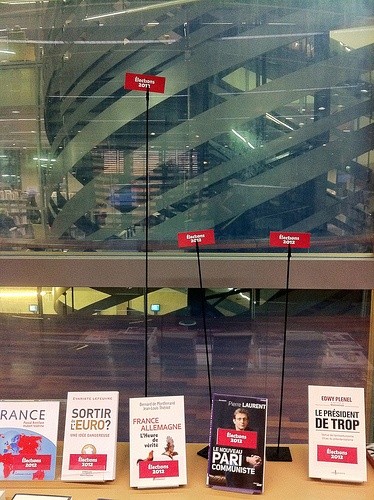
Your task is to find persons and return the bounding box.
[219,408,262,490]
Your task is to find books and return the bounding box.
[206,393,268,495]
[308,384,367,483]
[367,445,374,468]
[60,391,119,481]
[0,402,60,481]
[129,395,187,487]
